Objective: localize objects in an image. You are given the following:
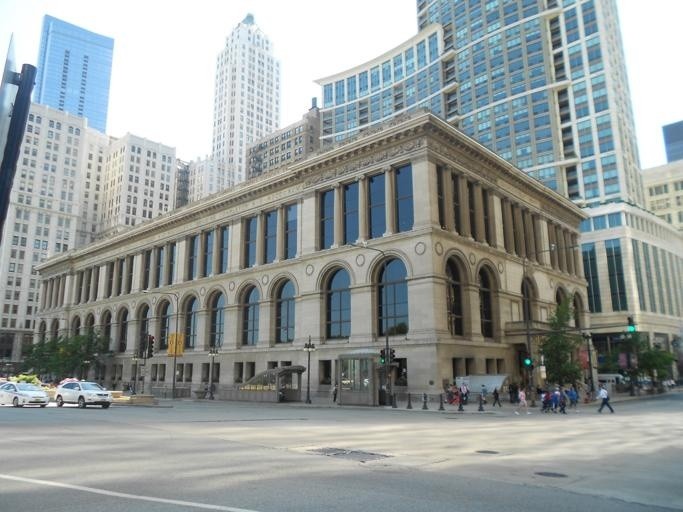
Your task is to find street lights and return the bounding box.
[205,344,219,401]
[304,336,316,403]
[523,244,580,398]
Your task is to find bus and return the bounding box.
[597,374,624,384]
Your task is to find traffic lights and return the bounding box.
[380,349,386,363]
[522,357,532,369]
[147,335,154,358]
[626,317,636,337]
[390,350,395,363]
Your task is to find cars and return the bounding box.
[54,381,114,409]
[0,381,50,408]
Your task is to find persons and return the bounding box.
[446,381,613,415]
